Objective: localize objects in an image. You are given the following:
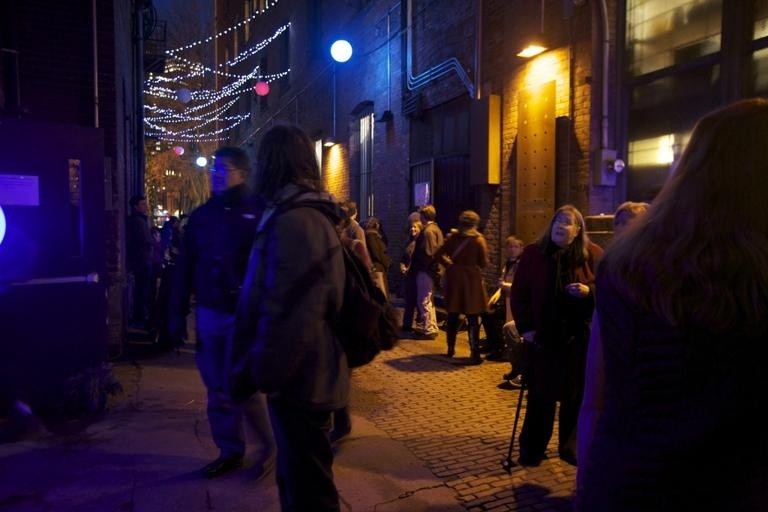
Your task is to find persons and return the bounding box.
[328,99,768,511]
[161,147,277,482]
[127,195,156,333]
[227,125,350,512]
[159,217,176,258]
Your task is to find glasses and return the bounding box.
[553,217,578,227]
[207,167,238,176]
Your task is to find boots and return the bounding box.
[468,326,483,363]
[446,324,456,358]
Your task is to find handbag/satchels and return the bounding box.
[440,272,446,287]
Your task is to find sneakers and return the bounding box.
[413,333,436,340]
[202,457,245,480]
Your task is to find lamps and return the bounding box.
[323,135,339,148]
[514,0,551,59]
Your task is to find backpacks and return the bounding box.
[340,242,401,369]
